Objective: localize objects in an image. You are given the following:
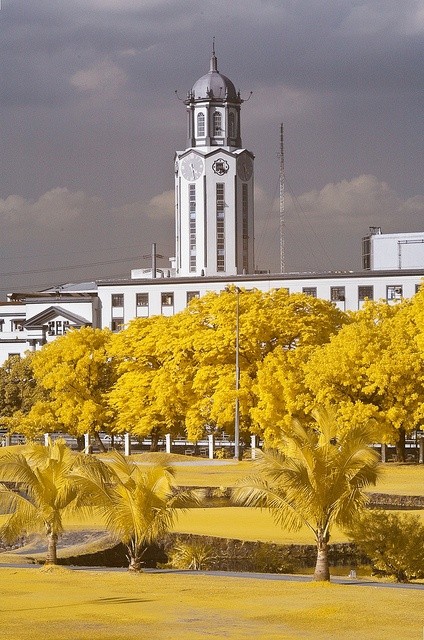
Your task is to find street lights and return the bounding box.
[225,287,245,462]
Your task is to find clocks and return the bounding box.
[181,154,204,181]
[236,154,254,184]
